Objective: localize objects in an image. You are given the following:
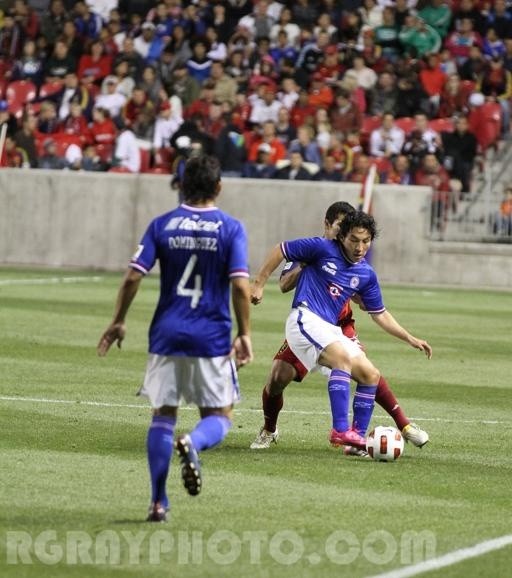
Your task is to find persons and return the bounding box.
[1,0,510,243]
[95,153,254,525]
[249,200,430,449]
[250,209,435,459]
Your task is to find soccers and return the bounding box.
[365,425,405,464]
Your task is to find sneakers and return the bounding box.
[147,503,166,522]
[329,428,367,457]
[174,434,202,495]
[250,427,279,450]
[402,421,429,449]
